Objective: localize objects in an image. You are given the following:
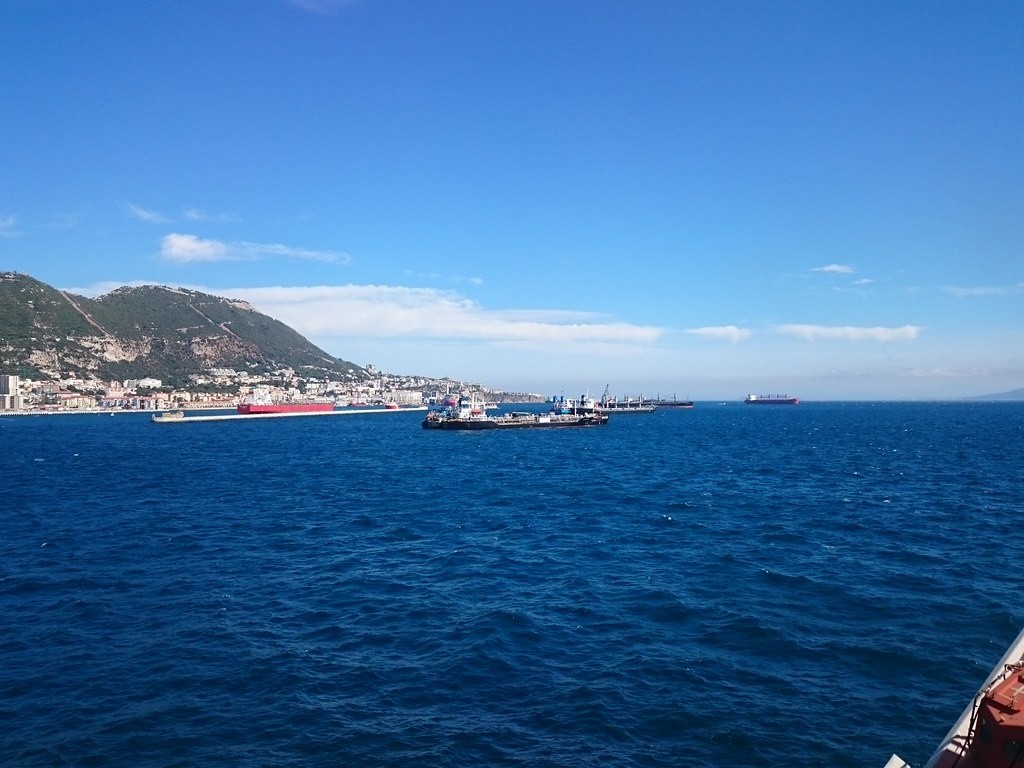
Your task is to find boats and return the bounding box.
[151,408,184,422]
[744,392,799,405]
[236,381,338,415]
[421,383,694,431]
